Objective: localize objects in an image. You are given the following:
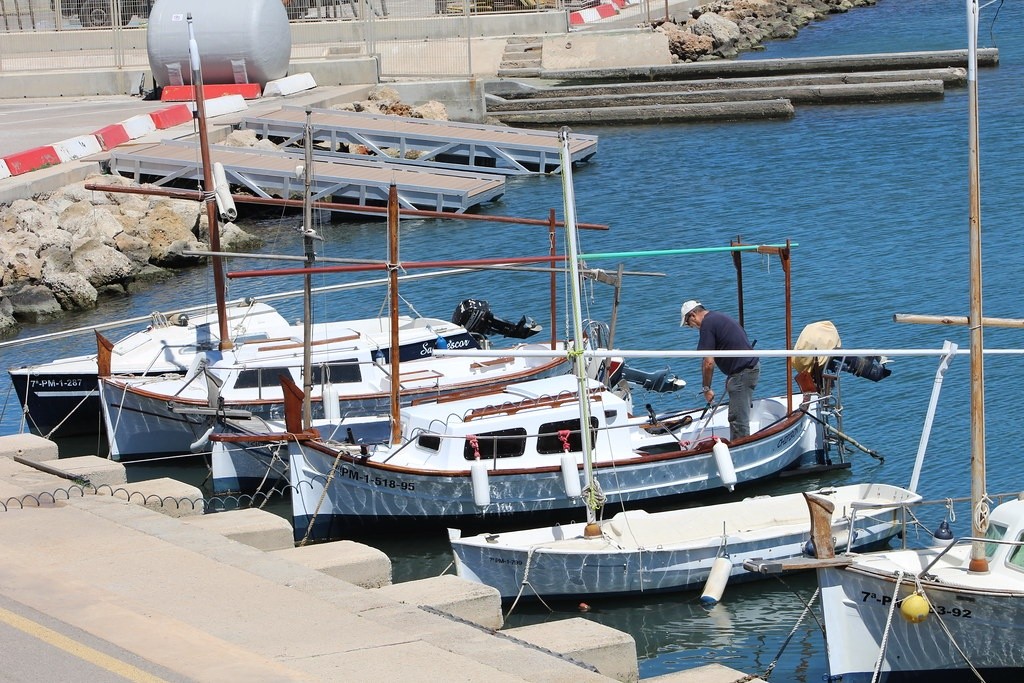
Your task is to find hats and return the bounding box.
[680,300,701,328]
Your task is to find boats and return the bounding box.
[445,123,962,601]
[741,0,1024,683]
[287,185,894,548]
[92,11,545,467]
[7,300,290,440]
[202,109,686,512]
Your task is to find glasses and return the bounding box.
[685,314,691,325]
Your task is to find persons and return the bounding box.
[680,301,760,441]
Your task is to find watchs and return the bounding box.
[702,386,711,392]
